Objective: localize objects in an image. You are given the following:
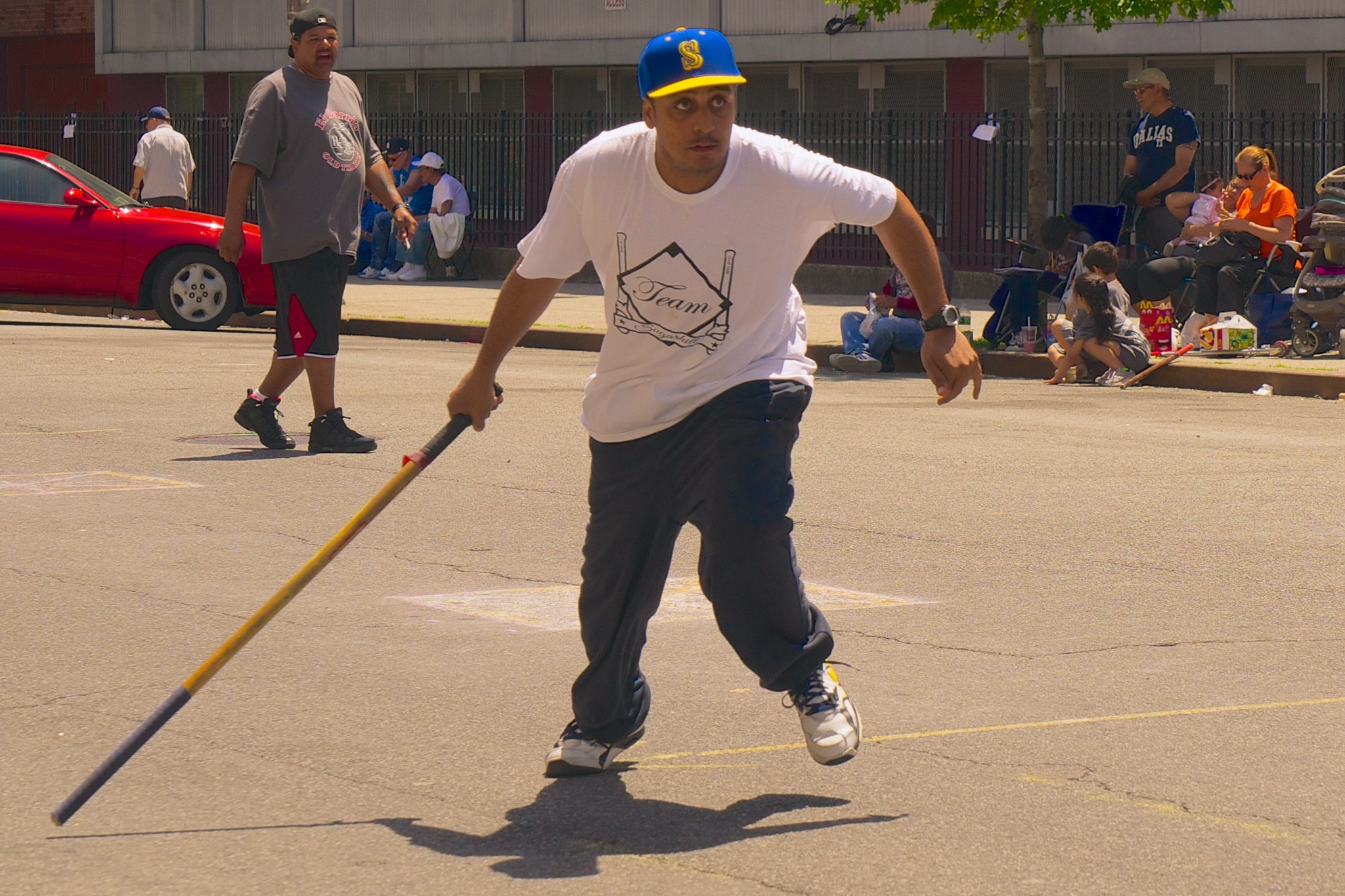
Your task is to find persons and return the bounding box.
[1042,272,1152,389]
[216,6,420,454]
[1113,68,1202,261]
[357,136,434,279]
[444,22,985,780]
[1047,239,1132,383]
[343,192,388,277]
[829,209,956,377]
[387,152,472,283]
[128,107,197,211]
[1193,143,1299,343]
[1040,212,1097,294]
[1162,169,1234,258]
[1137,172,1280,332]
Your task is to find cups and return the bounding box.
[1021,326,1037,353]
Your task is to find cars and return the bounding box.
[0,144,276,332]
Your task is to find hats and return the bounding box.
[381,138,410,155]
[412,152,445,170]
[1123,68,1170,91]
[288,7,337,59]
[141,106,172,121]
[638,27,747,100]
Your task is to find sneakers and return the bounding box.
[358,266,380,278]
[400,270,427,281]
[837,353,882,373]
[377,267,394,280]
[546,719,645,777]
[781,659,864,766]
[233,388,296,449]
[308,407,377,453]
[388,267,411,280]
[830,354,844,371]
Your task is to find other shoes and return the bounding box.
[1055,364,1094,383]
[1095,368,1117,384]
[1101,369,1139,386]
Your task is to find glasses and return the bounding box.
[1134,84,1157,95]
[387,152,404,161]
[1236,165,1263,180]
[1230,182,1245,192]
[1214,188,1222,192]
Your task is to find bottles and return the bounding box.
[957,303,970,345]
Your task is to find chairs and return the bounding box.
[422,192,479,282]
[979,202,1129,348]
[1237,208,1311,321]
[1135,205,1209,314]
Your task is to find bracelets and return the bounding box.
[132,185,140,190]
[364,230,369,240]
[391,201,413,215]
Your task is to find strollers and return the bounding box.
[1291,162,1345,358]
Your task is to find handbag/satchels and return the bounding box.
[1195,230,1261,266]
